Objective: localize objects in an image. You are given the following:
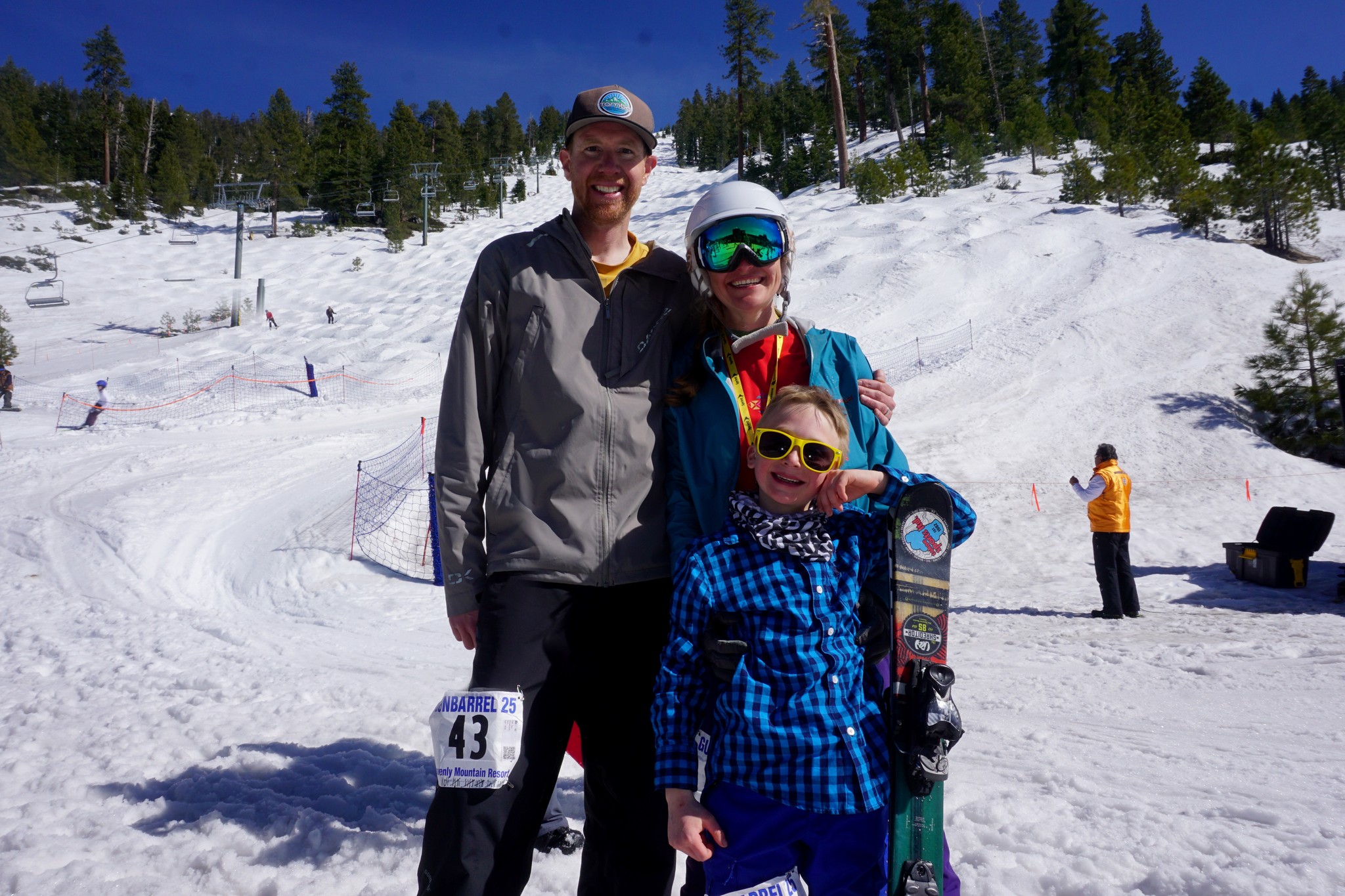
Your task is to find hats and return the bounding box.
[0,365,5,371]
[565,84,657,155]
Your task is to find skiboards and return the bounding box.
[891,482,954,896]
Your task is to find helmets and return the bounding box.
[96,380,106,388]
[684,181,796,299]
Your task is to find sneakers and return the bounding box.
[1125,610,1140,617]
[1090,610,1124,620]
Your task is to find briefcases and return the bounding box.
[1223,508,1334,589]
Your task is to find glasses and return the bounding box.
[1094,455,1099,458]
[693,215,789,273]
[97,385,102,387]
[752,428,842,473]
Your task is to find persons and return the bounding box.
[1069,443,1140,619]
[663,180,908,577]
[326,306,337,324]
[0,364,13,407]
[648,386,976,896]
[82,380,110,428]
[266,310,278,330]
[413,84,896,896]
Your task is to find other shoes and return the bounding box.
[536,827,586,855]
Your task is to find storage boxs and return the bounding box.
[1223,507,1335,587]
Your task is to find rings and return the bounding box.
[884,407,889,416]
